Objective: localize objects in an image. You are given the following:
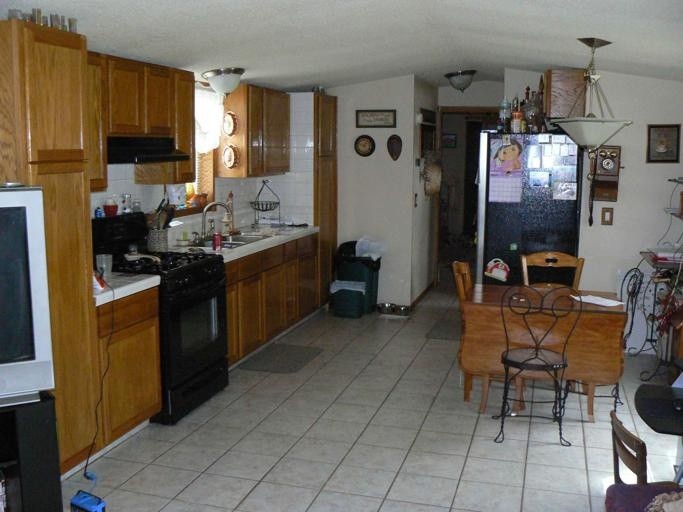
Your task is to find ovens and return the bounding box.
[151,274,231,426]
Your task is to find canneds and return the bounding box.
[213,233,224,251]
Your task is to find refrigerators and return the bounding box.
[476,130,582,287]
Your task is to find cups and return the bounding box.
[96,255,113,276]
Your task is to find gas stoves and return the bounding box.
[118,251,223,290]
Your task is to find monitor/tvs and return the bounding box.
[0,185,56,409]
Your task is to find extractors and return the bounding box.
[107,136,191,171]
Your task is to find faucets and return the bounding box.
[202,201,231,240]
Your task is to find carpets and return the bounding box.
[426,305,462,341]
[238,342,323,374]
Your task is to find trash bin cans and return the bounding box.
[330,241,381,317]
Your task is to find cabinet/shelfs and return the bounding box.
[224,234,321,369]
[256,92,337,308]
[0,18,97,481]
[213,82,291,178]
[97,286,163,448]
[88,51,196,193]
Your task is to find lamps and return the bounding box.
[550,36,633,154]
[201,67,245,100]
[444,70,477,93]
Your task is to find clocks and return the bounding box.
[354,135,375,157]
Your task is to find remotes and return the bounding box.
[3,181,22,188]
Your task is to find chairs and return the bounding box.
[605,411,680,485]
[452,250,643,446]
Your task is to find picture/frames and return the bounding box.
[356,109,396,128]
[443,134,457,148]
[647,124,680,164]
[419,124,436,158]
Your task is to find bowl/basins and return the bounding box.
[379,302,410,314]
[102,204,118,215]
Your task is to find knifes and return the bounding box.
[155,198,174,228]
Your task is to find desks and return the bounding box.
[635,384,682,484]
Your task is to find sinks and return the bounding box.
[188,242,243,250]
[222,235,272,241]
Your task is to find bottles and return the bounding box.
[497,75,546,133]
[8,6,79,34]
[119,194,141,213]
